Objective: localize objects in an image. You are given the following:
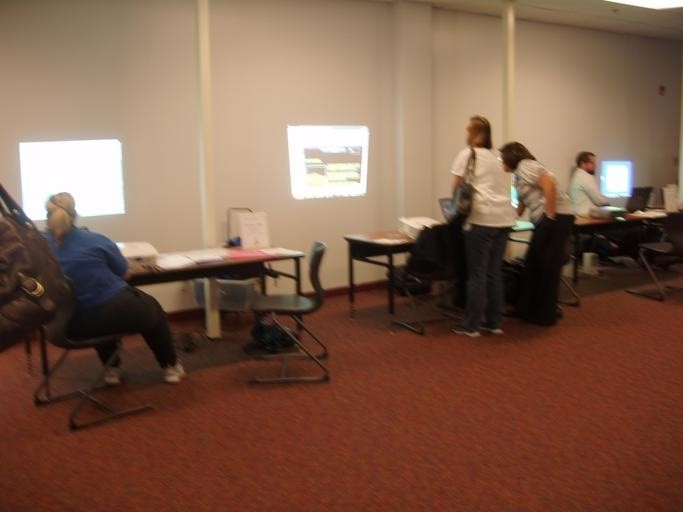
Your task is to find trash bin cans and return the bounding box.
[583,252,599,276]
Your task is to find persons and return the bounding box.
[38,193,185,384]
[500,142,576,327]
[569,152,644,269]
[449,116,512,339]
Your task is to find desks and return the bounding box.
[343,220,535,314]
[21,245,306,398]
[565,207,667,285]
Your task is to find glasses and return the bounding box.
[586,159,596,164]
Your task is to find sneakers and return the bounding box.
[101,366,126,384]
[478,324,504,335]
[165,362,185,382]
[451,325,480,337]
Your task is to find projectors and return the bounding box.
[117,240,158,271]
[591,205,626,219]
[398,216,441,240]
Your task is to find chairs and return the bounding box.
[248,240,330,384]
[33,275,153,429]
[625,212,683,301]
[386,224,465,335]
[626,186,657,213]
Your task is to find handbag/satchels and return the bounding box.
[438,149,475,224]
[0,185,73,353]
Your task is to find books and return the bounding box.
[638,210,667,219]
[218,247,268,261]
[262,246,302,257]
[186,250,223,264]
[155,252,193,272]
[399,216,443,241]
